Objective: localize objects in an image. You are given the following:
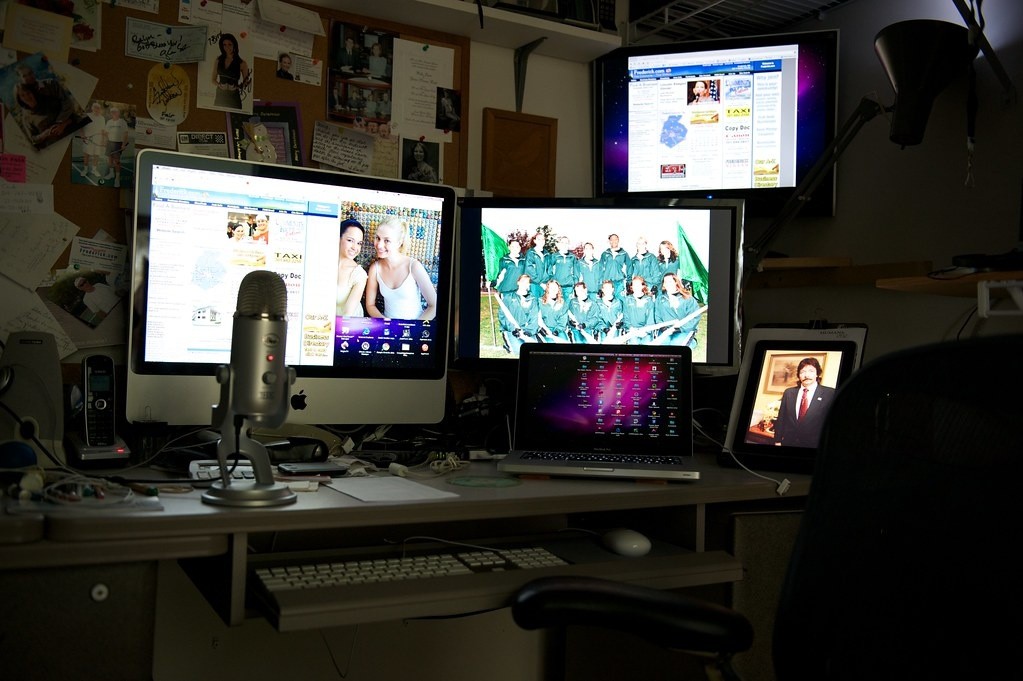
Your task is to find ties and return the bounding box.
[798,389,809,424]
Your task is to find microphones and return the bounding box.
[228,271,287,421]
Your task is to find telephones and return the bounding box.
[76,354,132,460]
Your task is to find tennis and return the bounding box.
[99,178,105,185]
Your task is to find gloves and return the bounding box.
[490,280,497,287]
[597,290,603,298]
[651,286,657,295]
[509,320,681,340]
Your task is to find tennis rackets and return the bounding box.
[75,134,109,147]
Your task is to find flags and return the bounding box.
[482,225,510,282]
[679,225,709,305]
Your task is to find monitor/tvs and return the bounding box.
[125,27,842,460]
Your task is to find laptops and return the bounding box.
[498,344,709,485]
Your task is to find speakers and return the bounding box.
[0,330,67,469]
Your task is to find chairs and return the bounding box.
[510,330,1023,681]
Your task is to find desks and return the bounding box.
[0,446,819,681]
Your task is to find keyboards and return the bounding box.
[254,546,577,631]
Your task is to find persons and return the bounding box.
[252,214,268,245]
[211,34,251,109]
[336,219,369,317]
[329,87,391,120]
[230,224,244,241]
[246,216,257,237]
[228,221,235,238]
[13,64,88,149]
[103,108,129,186]
[80,102,106,177]
[277,54,294,81]
[366,216,436,321]
[369,43,387,79]
[494,233,699,352]
[73,269,122,317]
[773,358,835,449]
[337,37,362,71]
[688,82,715,105]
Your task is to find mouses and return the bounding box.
[597,527,651,558]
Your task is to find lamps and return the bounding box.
[744,20,981,307]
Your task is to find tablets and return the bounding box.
[731,339,858,462]
